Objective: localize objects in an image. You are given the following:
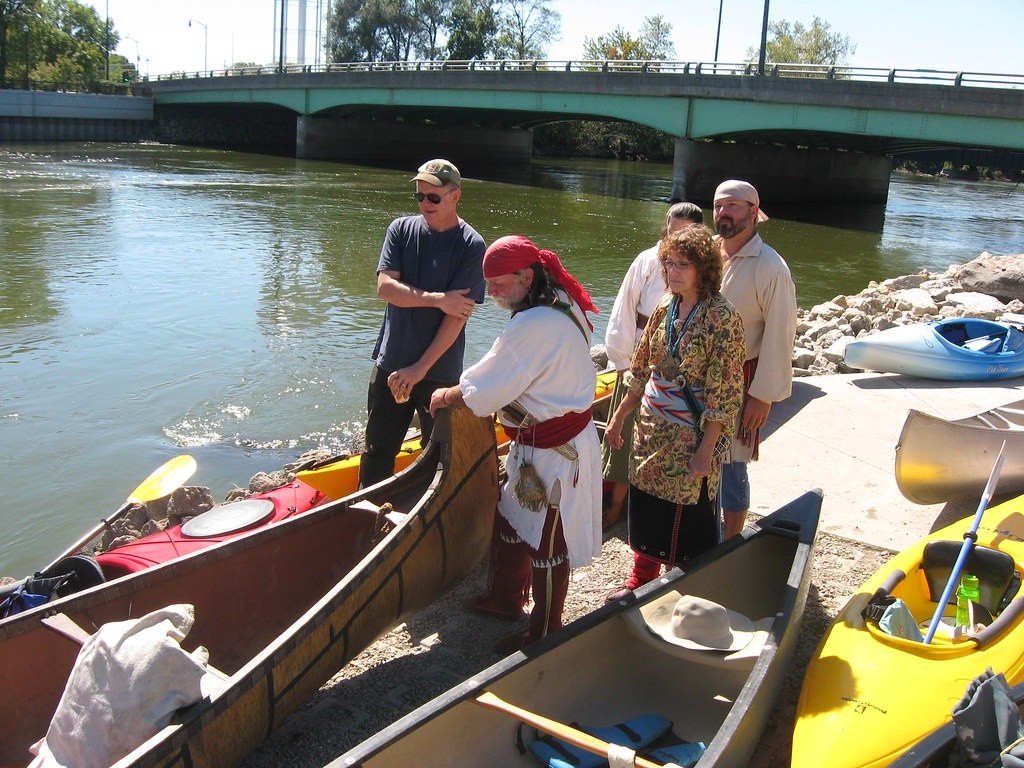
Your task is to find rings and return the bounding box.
[402,382,406,387]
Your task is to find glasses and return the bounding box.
[414,192,455,204]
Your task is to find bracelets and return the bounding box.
[443,390,453,405]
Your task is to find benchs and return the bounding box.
[623,589,775,671]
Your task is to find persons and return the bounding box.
[605,224,748,606]
[357,158,486,492]
[601,203,704,532]
[429,234,602,655]
[710,180,798,540]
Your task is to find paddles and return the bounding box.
[40,453,198,579]
[924,438,1009,648]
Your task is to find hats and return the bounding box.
[409,159,462,190]
[713,177,768,223]
[646,595,757,651]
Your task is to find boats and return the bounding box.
[892,401,1024,505]
[787,493,1024,767]
[304,486,816,767]
[0,390,494,768]
[841,317,1024,380]
[0,354,616,619]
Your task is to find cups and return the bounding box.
[955,574,980,627]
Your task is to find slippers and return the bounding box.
[601,509,617,529]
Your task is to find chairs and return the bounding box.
[904,539,1016,646]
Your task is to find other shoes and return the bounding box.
[605,590,633,604]
[494,629,534,654]
[466,591,521,622]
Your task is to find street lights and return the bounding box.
[189,19,208,76]
[124,35,140,79]
